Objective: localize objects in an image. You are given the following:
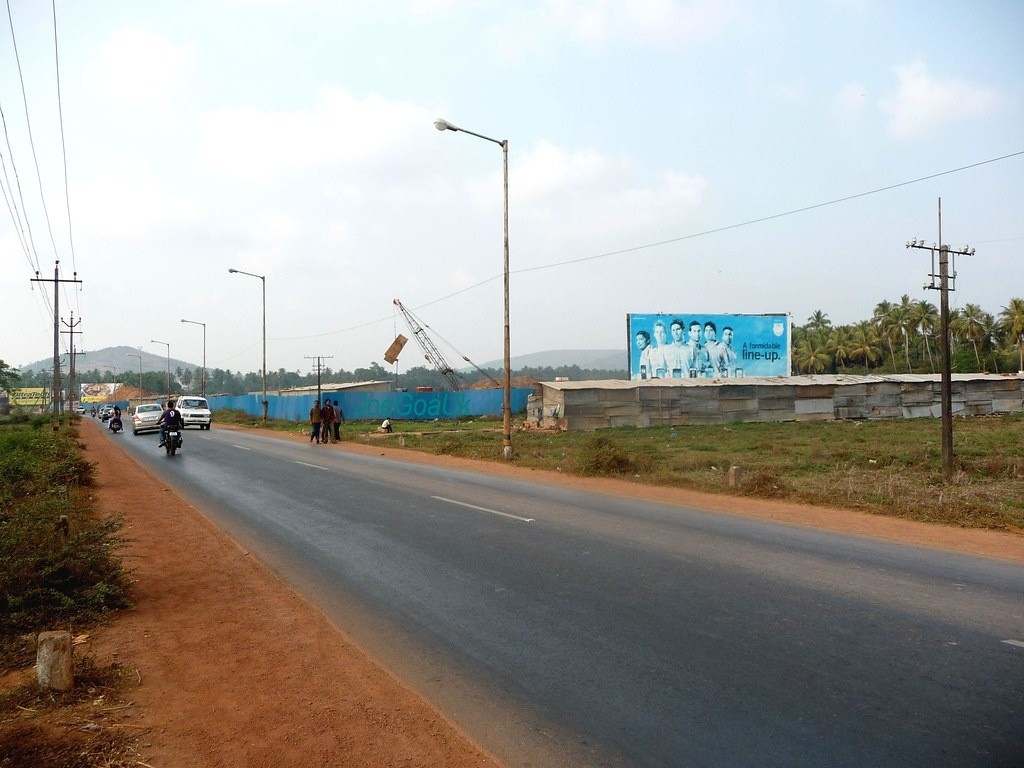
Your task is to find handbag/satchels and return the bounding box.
[331,414,336,424]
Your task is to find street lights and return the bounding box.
[228,268,269,422]
[101,365,116,407]
[151,339,171,402]
[180,319,206,398]
[433,117,514,454]
[126,354,142,406]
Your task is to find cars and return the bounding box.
[133,403,165,435]
[101,408,112,422]
[98,405,112,419]
[76,406,87,415]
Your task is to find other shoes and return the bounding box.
[158,441,165,447]
[332,440,337,444]
[310,435,314,440]
[316,439,319,444]
[323,439,328,444]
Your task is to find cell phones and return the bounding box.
[639,364,745,380]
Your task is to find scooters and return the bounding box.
[90,410,97,417]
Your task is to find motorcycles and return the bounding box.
[157,423,184,455]
[109,417,120,433]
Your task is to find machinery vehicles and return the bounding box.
[395,298,463,390]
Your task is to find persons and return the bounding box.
[382,417,394,433]
[648,321,670,378]
[688,321,716,373]
[319,399,337,444]
[703,322,720,355]
[156,401,182,448]
[309,400,321,444]
[127,405,130,415]
[661,319,701,379]
[715,327,745,377]
[90,406,96,415]
[333,400,345,442]
[106,406,122,431]
[636,330,653,380]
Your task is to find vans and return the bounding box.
[174,396,212,431]
